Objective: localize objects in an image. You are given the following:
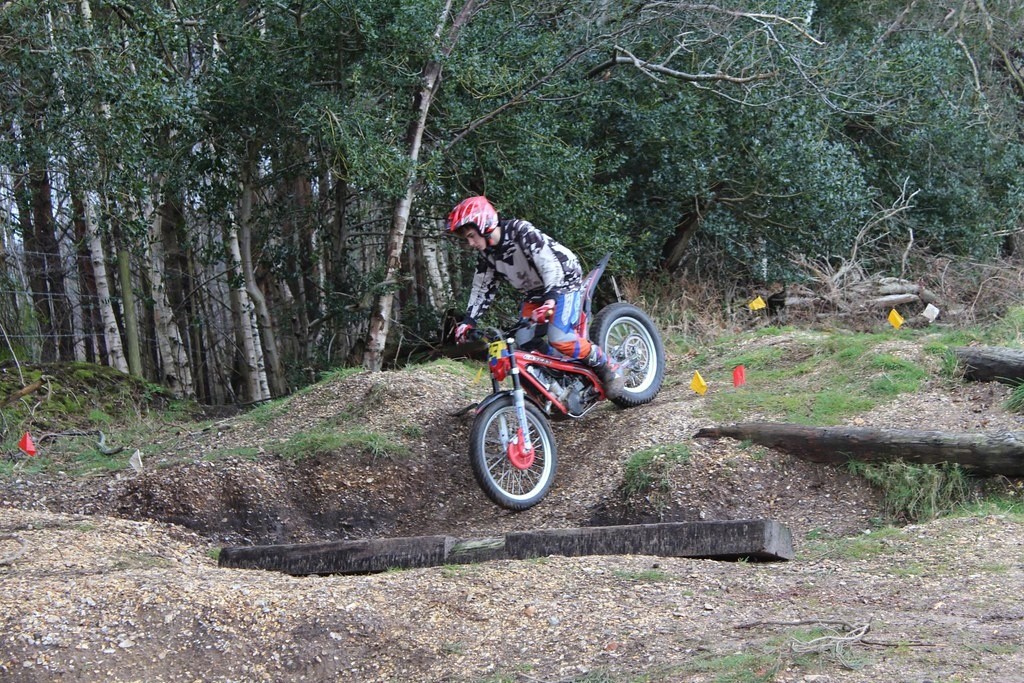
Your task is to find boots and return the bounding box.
[581,339,624,399]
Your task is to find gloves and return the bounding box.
[455,323,474,344]
[531,304,556,325]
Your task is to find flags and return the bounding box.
[732,365,745,387]
[887,308,904,331]
[689,371,708,397]
[921,303,940,323]
[748,296,766,311]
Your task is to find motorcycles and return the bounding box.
[448,252,666,514]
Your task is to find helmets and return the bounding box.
[446,196,499,237]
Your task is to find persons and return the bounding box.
[446,195,627,399]
